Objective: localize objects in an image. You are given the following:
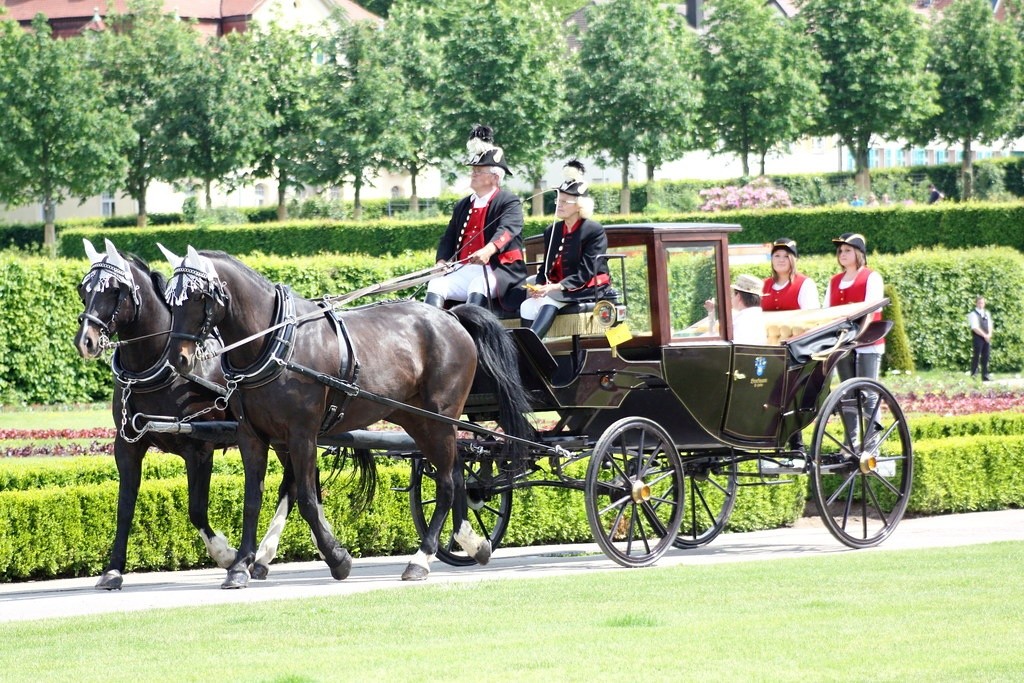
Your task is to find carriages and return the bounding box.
[71,217,915,592]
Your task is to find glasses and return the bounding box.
[554,198,576,207]
[470,169,495,176]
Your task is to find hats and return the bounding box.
[832,233,865,255]
[551,159,589,197]
[465,123,513,176]
[772,238,797,258]
[730,275,770,296]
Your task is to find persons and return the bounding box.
[703,275,767,344]
[521,159,610,341]
[849,184,944,206]
[823,233,885,458]
[968,296,996,382]
[424,123,528,307]
[760,237,821,467]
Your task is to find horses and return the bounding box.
[151,238,541,590]
[71,235,378,589]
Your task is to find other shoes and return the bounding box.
[756,456,787,471]
[785,453,808,468]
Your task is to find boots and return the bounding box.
[424,292,445,310]
[465,292,488,308]
[531,304,559,340]
[520,316,534,328]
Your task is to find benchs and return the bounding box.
[442,254,627,376]
[767,315,841,344]
